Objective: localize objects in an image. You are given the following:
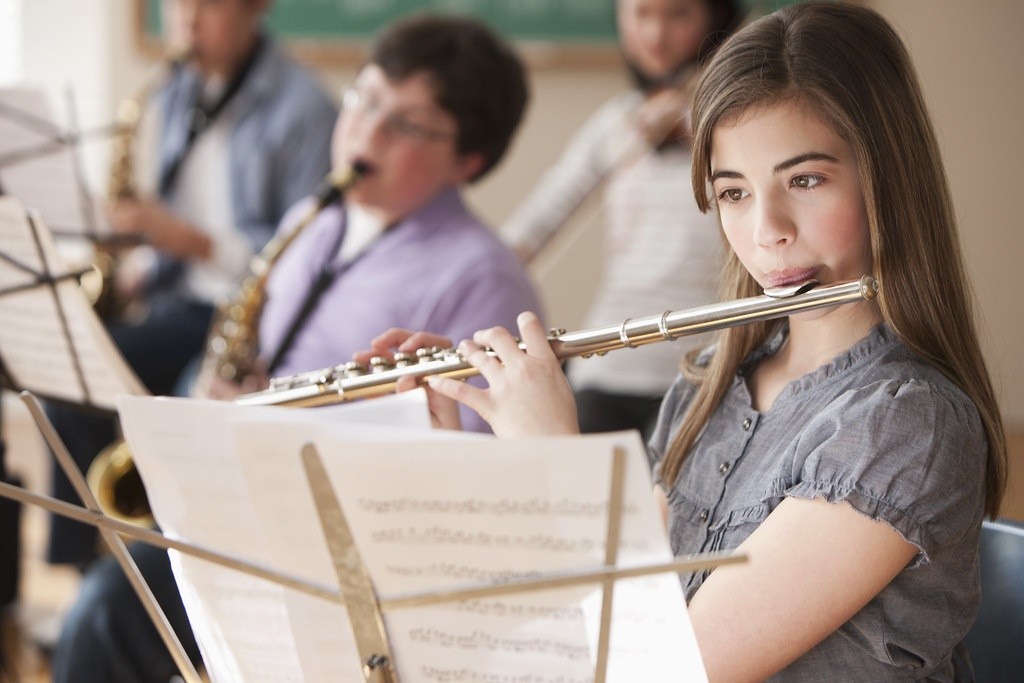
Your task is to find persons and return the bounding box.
[0,1,343,662]
[52,14,550,683]
[494,0,754,433]
[350,1,1010,683]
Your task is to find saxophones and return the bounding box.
[83,162,370,532]
[74,43,190,320]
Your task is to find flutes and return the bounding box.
[229,274,881,414]
